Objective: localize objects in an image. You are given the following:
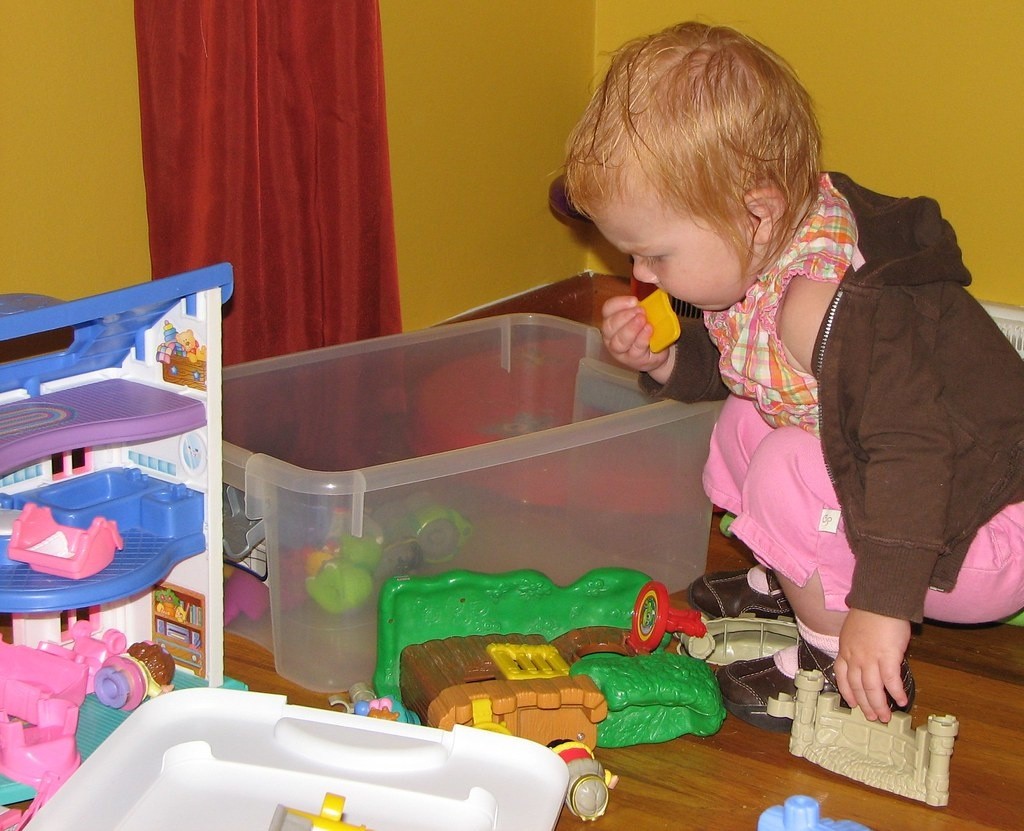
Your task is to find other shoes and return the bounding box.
[687,566,794,620]
[715,634,917,732]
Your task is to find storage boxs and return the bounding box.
[221,313,728,694]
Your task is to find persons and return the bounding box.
[545,20,1024,735]
